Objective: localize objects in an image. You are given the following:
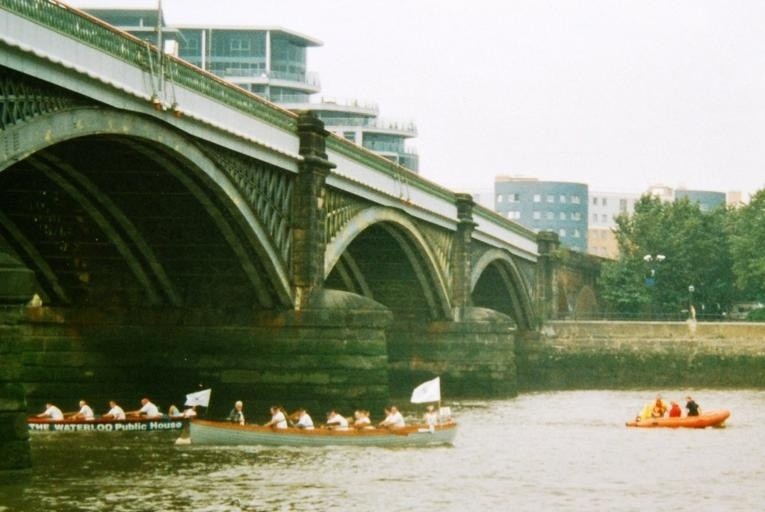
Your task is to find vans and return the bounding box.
[729,302,763,320]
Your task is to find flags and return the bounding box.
[184,388,211,407]
[409,375,441,404]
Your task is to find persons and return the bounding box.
[262,404,406,430]
[422,404,437,434]
[226,400,245,425]
[669,400,681,418]
[651,393,669,417]
[686,397,702,415]
[66,399,95,420]
[130,398,163,418]
[35,401,64,420]
[168,404,198,418]
[100,400,126,419]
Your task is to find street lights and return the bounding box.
[643,254,666,321]
[688,285,695,320]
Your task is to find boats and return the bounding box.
[185,415,460,447]
[625,409,732,428]
[27,409,194,443]
